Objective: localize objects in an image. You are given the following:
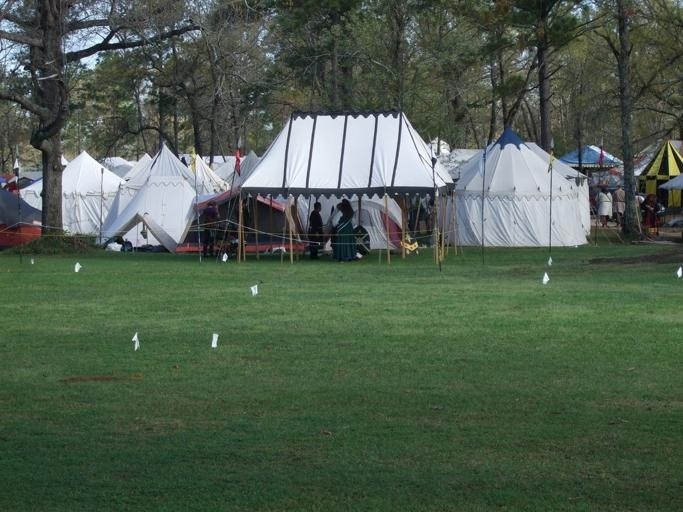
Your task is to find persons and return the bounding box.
[330,200,357,261]
[308,202,324,258]
[594,184,612,226]
[612,185,625,226]
[635,191,660,228]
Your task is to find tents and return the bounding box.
[434,128,591,247]
[0,142,260,253]
[611,140,681,209]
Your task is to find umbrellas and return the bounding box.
[556,144,623,168]
